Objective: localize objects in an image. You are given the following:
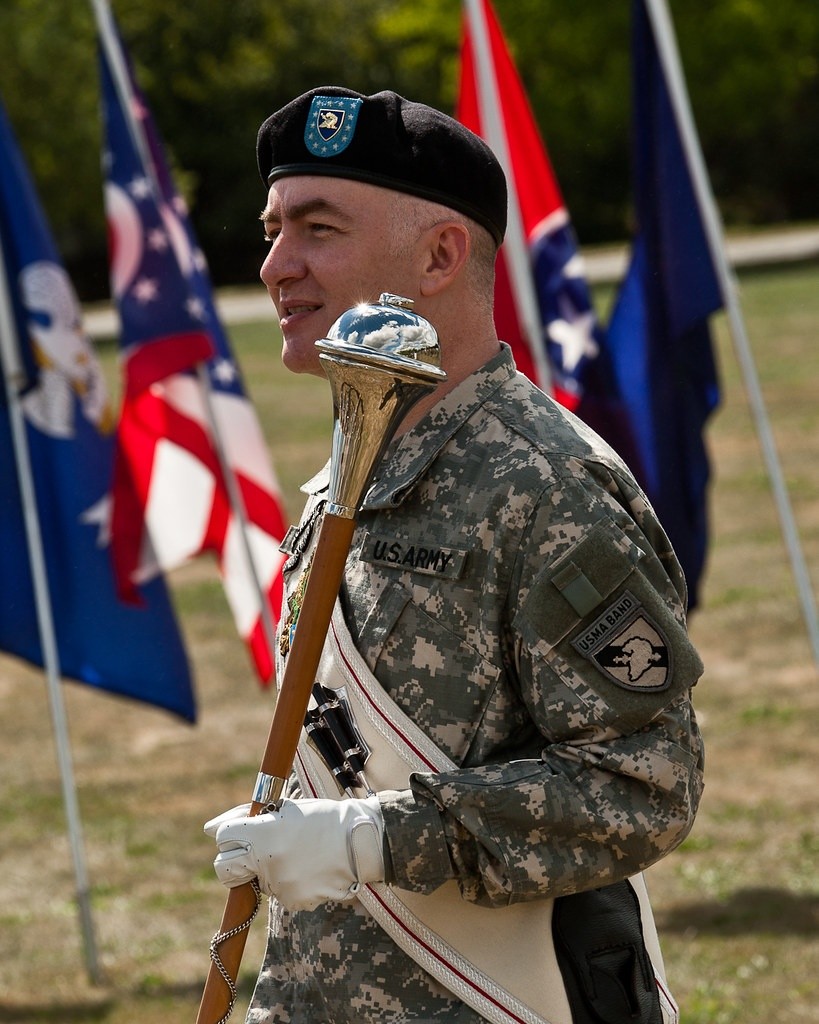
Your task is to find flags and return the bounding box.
[0,103,196,724]
[449,1,725,615]
[97,11,287,691]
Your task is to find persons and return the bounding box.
[204,86,704,1024]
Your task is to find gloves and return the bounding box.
[204,793,385,911]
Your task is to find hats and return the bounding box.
[257,85,509,245]
